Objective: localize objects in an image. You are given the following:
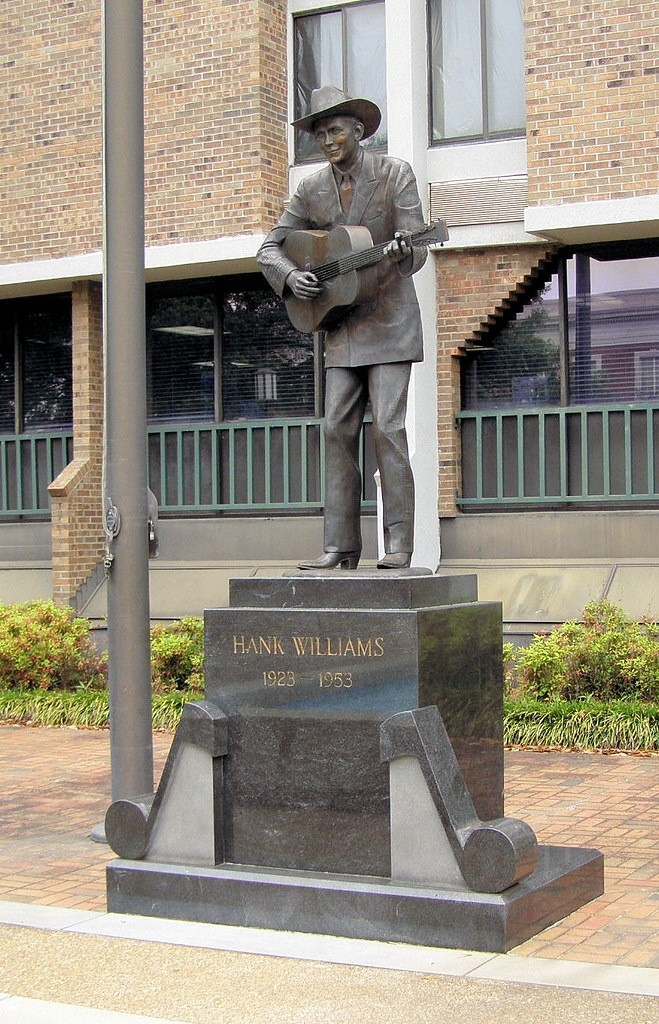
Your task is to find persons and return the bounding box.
[255,85,428,570]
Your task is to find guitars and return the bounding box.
[281,218,449,334]
